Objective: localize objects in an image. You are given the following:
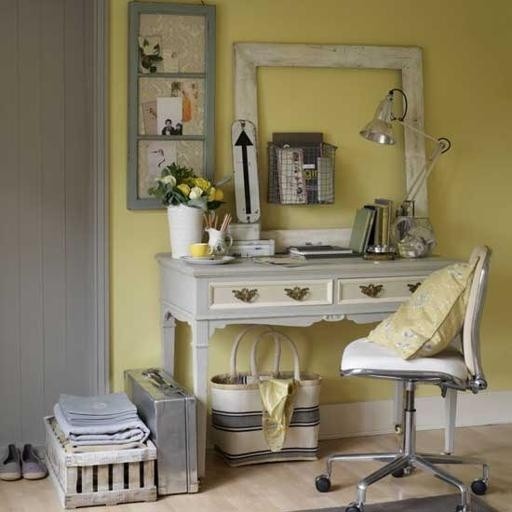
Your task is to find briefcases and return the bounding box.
[124,366,199,495]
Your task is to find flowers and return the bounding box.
[148,161,226,213]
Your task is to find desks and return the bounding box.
[156,254,458,484]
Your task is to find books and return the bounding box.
[349,204,387,255]
[287,244,352,256]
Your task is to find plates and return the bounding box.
[184,257,235,264]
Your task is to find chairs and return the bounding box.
[316,246,489,512]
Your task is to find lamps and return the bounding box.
[358,88,451,258]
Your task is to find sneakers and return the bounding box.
[22,444,49,481]
[1,443,23,481]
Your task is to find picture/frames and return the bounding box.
[126,1,216,210]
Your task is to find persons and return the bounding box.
[161,118,173,134]
[167,123,181,134]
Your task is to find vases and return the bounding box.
[167,204,205,259]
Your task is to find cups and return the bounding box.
[189,243,213,257]
[205,225,234,256]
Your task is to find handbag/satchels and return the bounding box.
[209,328,323,469]
